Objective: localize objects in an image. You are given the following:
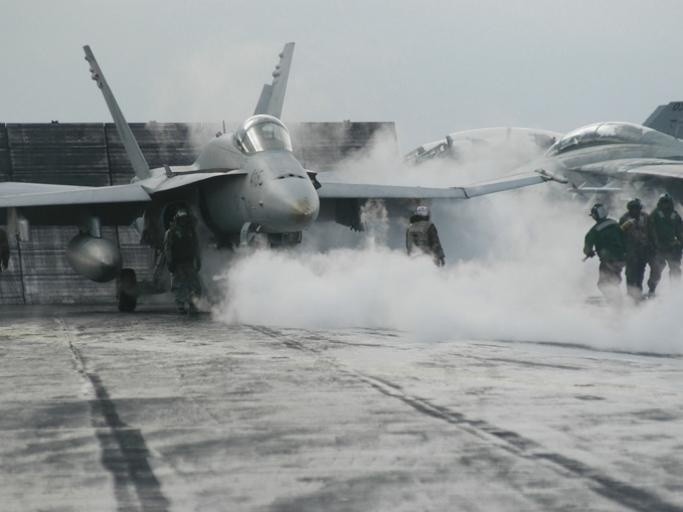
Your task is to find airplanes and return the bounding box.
[0,41,575,318]
[532,101,683,213]
[396,125,564,180]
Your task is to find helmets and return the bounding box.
[628,198,642,209]
[658,193,674,208]
[590,204,609,221]
[414,205,432,219]
[173,210,189,219]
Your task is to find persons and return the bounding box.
[160,207,203,316]
[404,205,446,269]
[581,202,625,300]
[646,192,682,301]
[617,198,651,306]
